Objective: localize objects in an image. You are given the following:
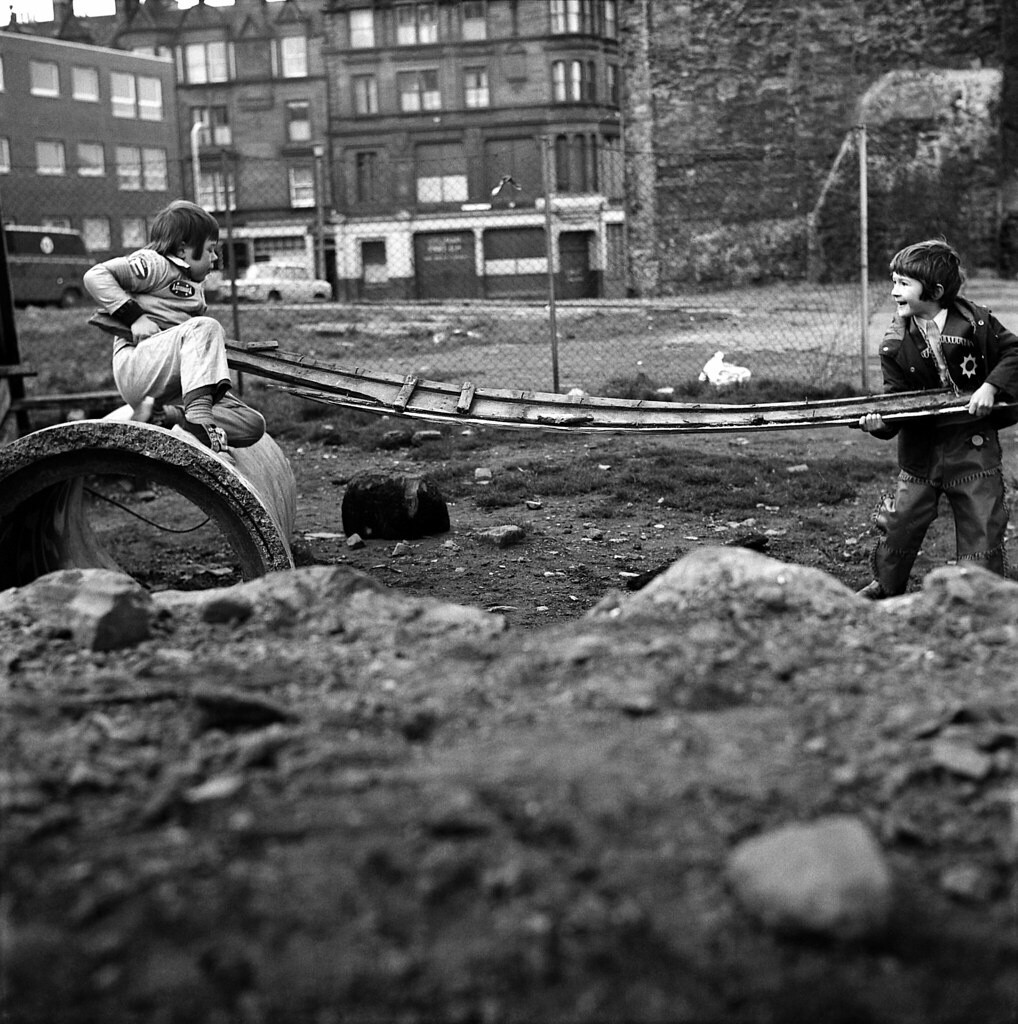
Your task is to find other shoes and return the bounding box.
[857,580,887,600]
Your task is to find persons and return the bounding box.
[81,199,266,465]
[848,239,1018,601]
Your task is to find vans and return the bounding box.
[4,224,95,308]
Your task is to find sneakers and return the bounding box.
[173,417,235,464]
[131,396,168,428]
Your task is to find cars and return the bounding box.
[220,263,332,305]
